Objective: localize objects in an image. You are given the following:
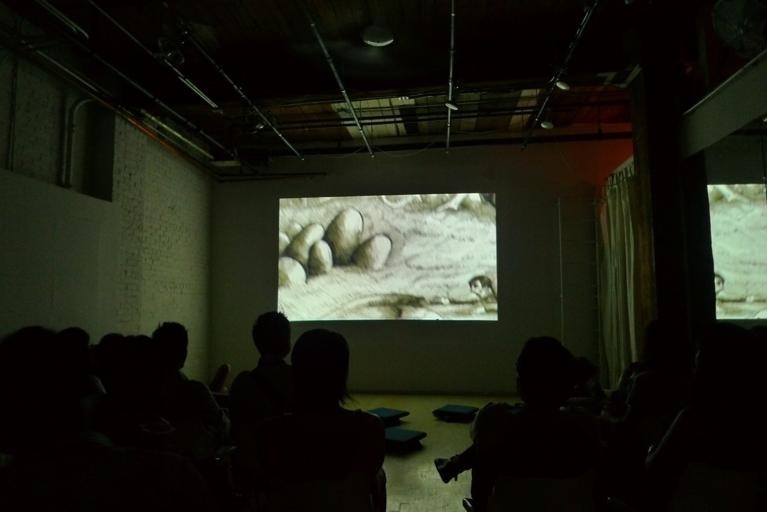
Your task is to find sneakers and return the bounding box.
[433,454,464,482]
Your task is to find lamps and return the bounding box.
[0,1,614,169]
